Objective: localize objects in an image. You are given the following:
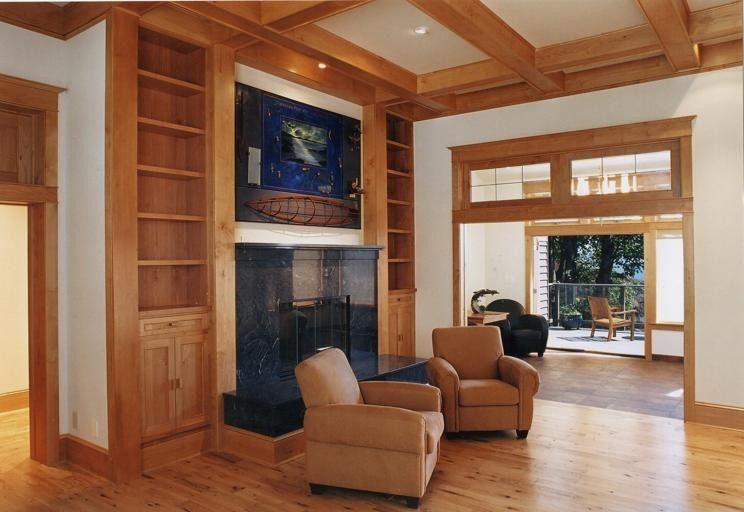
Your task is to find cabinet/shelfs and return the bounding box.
[102,7,215,479]
[360,102,414,364]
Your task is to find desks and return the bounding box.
[467,310,508,328]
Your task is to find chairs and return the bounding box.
[586,294,638,343]
[486,297,548,357]
[293,344,444,508]
[423,324,543,439]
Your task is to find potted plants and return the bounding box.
[557,302,585,329]
